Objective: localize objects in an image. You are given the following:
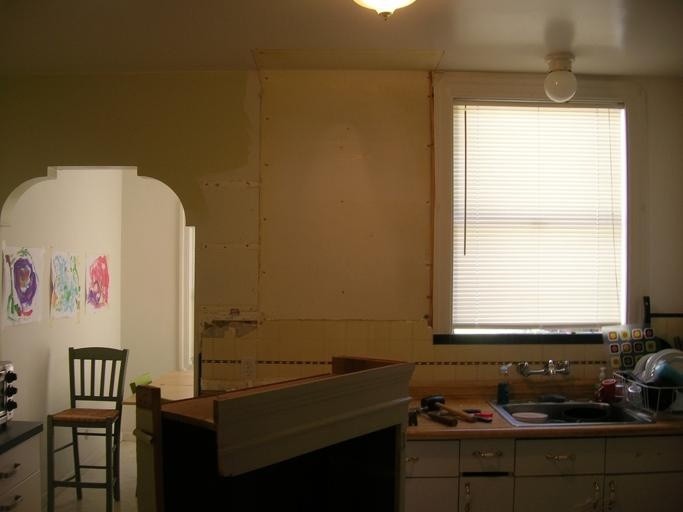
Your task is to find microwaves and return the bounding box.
[0,361,18,427]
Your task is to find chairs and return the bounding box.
[47,347,129,512]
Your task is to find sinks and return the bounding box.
[486,396,656,427]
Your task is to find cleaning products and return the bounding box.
[593,367,608,401]
[497,363,513,402]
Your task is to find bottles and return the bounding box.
[496,368,510,405]
[628,382,642,412]
[614,383,625,405]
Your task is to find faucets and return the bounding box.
[543,360,560,378]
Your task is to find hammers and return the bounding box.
[421,394,475,423]
[416,406,458,427]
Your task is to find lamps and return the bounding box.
[353,0,417,21]
[544,50,577,103]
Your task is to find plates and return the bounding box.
[511,411,549,424]
[634,347,682,412]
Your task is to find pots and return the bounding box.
[617,294,673,370]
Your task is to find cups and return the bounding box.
[595,378,616,403]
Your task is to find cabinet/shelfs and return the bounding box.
[0,433,41,512]
[515,435,683,512]
[399,439,515,512]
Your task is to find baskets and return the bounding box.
[612,370,683,421]
